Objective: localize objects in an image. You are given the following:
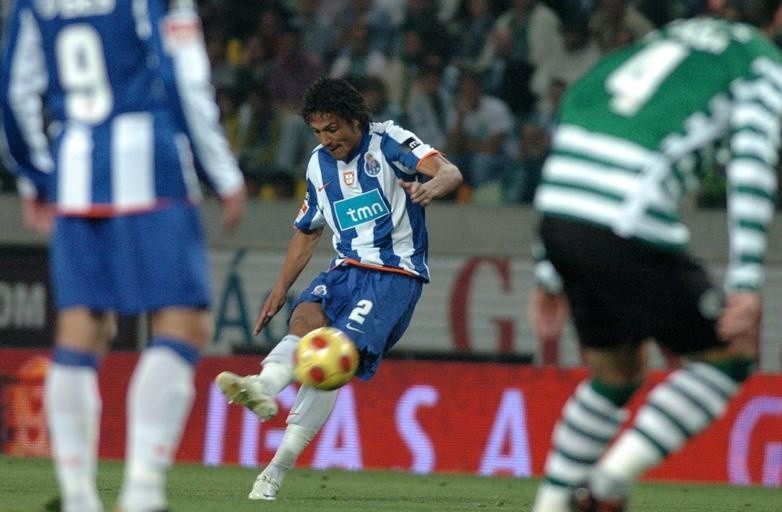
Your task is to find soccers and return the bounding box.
[293,326,358,389]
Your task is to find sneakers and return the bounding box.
[247,470,281,502]
[214,369,279,423]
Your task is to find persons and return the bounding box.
[0,0,250,512]
[531,0,782,512]
[214,75,464,503]
